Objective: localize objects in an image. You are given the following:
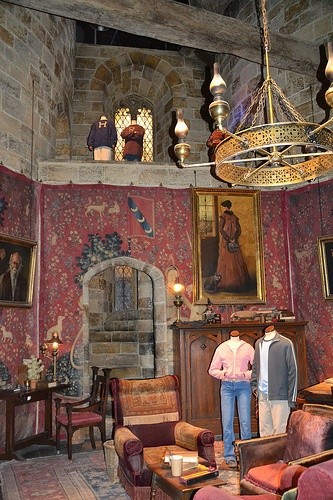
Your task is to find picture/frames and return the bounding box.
[317,235,333,302]
[191,187,266,305]
[0,232,38,308]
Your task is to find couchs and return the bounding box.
[233,404,333,496]
[192,459,332,500]
[108,373,215,500]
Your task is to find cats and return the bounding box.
[204,273,222,294]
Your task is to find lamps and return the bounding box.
[40,333,63,384]
[173,278,184,324]
[174,0,333,187]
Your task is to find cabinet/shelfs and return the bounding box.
[168,320,310,436]
[0,382,70,459]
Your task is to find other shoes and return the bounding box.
[226,459,237,467]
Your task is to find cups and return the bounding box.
[171,455,183,476]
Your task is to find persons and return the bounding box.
[120,119,145,161]
[250,325,298,467]
[208,330,255,467]
[87,116,118,160]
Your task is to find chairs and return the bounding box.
[53,366,111,459]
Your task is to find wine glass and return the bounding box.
[24,380,31,393]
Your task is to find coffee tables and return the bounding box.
[147,460,227,500]
